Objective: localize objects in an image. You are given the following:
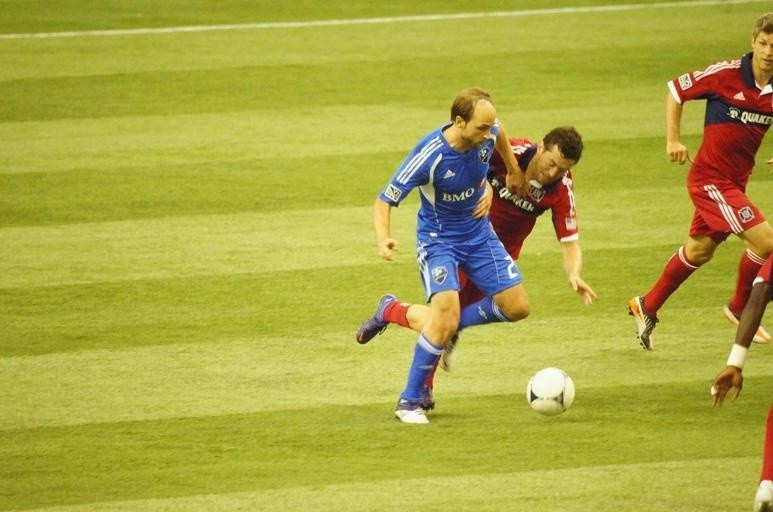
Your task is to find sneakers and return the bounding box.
[394,397,429,425]
[356,294,398,345]
[626,295,659,351]
[753,479,772,512]
[721,302,771,345]
[439,333,458,371]
[421,384,436,411]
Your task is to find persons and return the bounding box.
[709,253,772,512]
[623,7,771,352]
[369,84,536,425]
[354,123,598,411]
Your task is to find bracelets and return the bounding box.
[725,343,750,372]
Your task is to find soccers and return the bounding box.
[526,366,574,415]
